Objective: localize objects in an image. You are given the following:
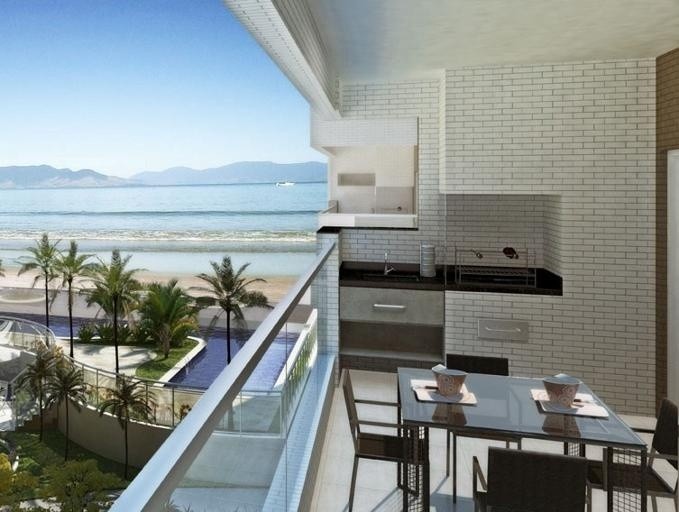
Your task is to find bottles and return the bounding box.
[503,246,519,260]
[420,245,435,278]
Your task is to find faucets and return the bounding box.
[384,253,394,276]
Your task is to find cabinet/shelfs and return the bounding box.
[336,284,444,379]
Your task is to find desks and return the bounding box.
[394,367,651,511]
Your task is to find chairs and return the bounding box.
[443,352,524,504]
[471,444,589,512]
[585,396,678,511]
[340,368,431,512]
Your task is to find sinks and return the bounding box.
[360,273,419,284]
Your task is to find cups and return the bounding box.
[542,376,582,409]
[433,369,468,397]
